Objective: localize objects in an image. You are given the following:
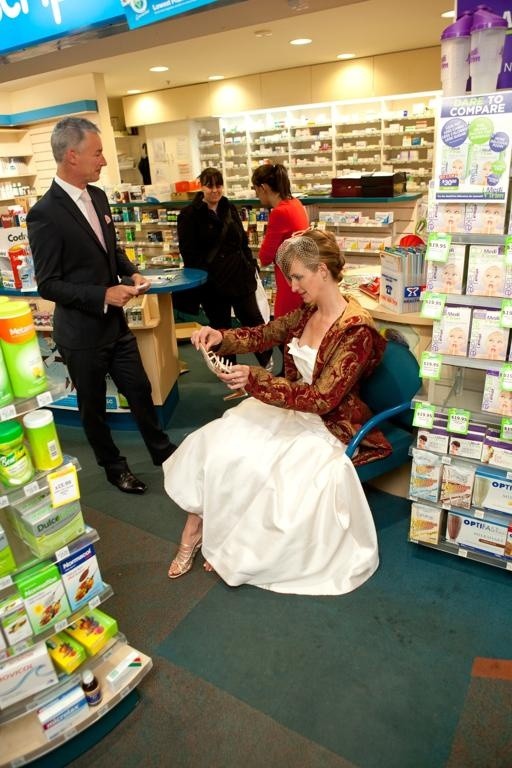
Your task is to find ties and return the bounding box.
[80,191,108,252]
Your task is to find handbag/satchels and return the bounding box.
[173,285,202,316]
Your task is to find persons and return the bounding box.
[26,118,179,494]
[252,164,309,377]
[42,333,75,392]
[177,169,274,373]
[139,144,151,184]
[419,160,512,464]
[161,228,392,596]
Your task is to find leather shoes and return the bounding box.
[107,470,148,494]
[151,443,179,466]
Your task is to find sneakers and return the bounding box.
[265,356,274,372]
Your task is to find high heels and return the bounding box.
[199,342,247,402]
[167,535,202,579]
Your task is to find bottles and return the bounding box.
[166,209,182,222]
[81,669,104,706]
[0,291,65,489]
[115,226,121,241]
[111,207,123,222]
